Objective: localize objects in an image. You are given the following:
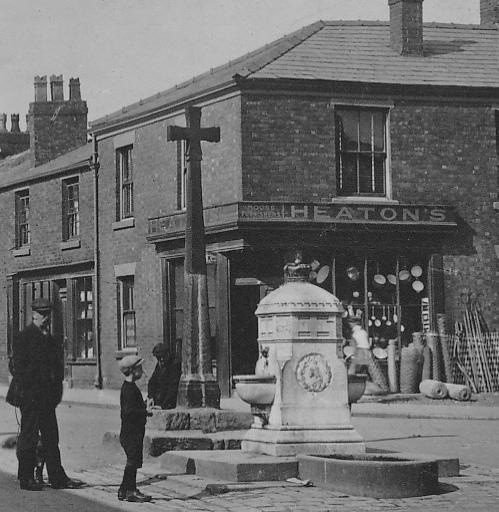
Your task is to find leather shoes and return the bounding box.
[127,488,151,502]
[21,479,42,490]
[52,478,86,489]
[118,491,127,500]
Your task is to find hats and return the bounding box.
[32,299,53,310]
[119,355,145,373]
[153,343,169,356]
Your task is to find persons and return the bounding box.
[344,316,376,383]
[114,353,155,503]
[3,297,89,491]
[140,342,181,411]
